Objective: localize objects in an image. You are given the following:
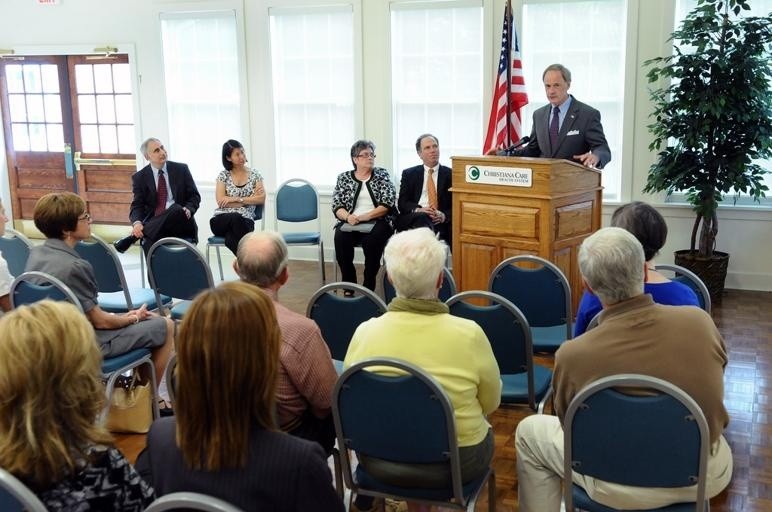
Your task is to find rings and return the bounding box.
[591,159,595,163]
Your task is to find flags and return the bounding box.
[482,0,528,155]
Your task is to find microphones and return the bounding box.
[504,136,530,152]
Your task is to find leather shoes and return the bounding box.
[113,236,134,254]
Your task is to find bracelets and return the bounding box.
[253,194,257,197]
[344,213,350,223]
[239,197,244,202]
[414,208,419,212]
[128,314,141,325]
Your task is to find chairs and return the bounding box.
[164,353,185,418]
[333,242,387,296]
[140,227,197,289]
[561,372,710,512]
[71,233,174,326]
[205,201,266,281]
[0,229,33,280]
[144,492,238,511]
[485,255,582,365]
[8,272,162,442]
[646,265,714,324]
[146,237,214,337]
[303,282,392,379]
[269,178,326,287]
[442,289,559,418]
[328,355,501,512]
[0,467,46,511]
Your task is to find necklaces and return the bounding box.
[648,267,656,271]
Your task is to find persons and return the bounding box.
[233,229,339,461]
[112,137,202,290]
[572,200,702,341]
[209,139,265,257]
[1,296,158,511]
[23,190,176,416]
[486,63,612,170]
[132,281,346,511]
[514,225,734,511]
[0,198,16,312]
[331,138,398,297]
[399,133,453,251]
[342,226,505,511]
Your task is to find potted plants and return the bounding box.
[640,1,772,304]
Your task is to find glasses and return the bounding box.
[357,152,376,158]
[78,212,90,221]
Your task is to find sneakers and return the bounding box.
[343,288,355,296]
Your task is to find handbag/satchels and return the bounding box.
[105,365,154,435]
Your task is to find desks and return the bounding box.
[449,156,604,320]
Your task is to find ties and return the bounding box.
[426,168,438,211]
[154,170,168,217]
[548,106,561,155]
